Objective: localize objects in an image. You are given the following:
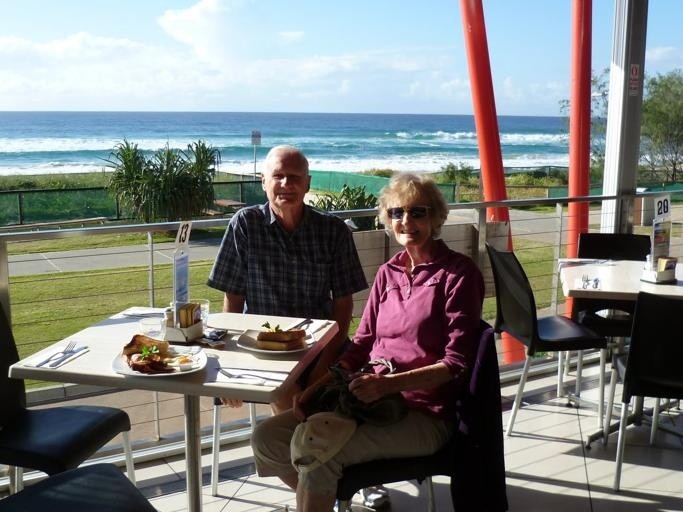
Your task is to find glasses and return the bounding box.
[386,206,431,219]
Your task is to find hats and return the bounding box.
[289,412,356,473]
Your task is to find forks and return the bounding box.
[215,367,284,382]
[37,341,77,368]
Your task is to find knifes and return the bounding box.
[50,346,88,368]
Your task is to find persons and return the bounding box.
[250,172,486,512]
[205,145,391,509]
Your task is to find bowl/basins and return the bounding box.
[139,317,164,337]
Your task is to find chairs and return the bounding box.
[1,302,138,496]
[612,291,683,493]
[0,464,157,512]
[211,393,256,497]
[565,233,663,418]
[336,318,498,512]
[482,242,611,437]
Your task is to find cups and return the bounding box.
[188,299,211,330]
[583,269,602,289]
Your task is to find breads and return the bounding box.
[257,330,307,351]
[123,334,169,373]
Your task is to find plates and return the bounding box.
[112,343,208,379]
[236,328,316,354]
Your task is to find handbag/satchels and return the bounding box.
[315,359,408,427]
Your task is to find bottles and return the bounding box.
[644,255,654,271]
[165,311,173,327]
